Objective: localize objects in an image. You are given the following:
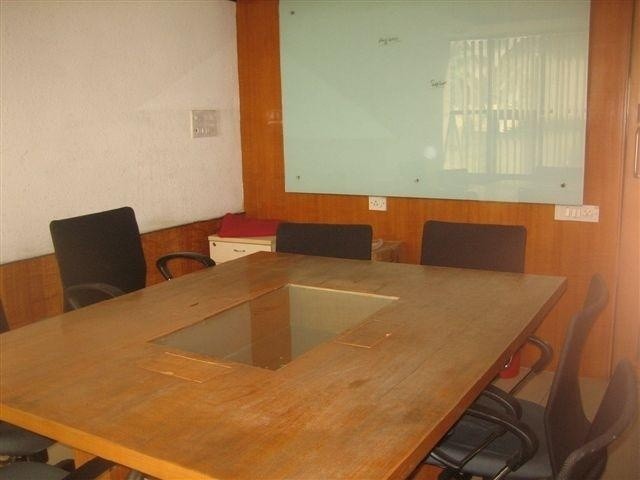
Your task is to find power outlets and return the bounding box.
[368,197,387,211]
[582,205,599,222]
[192,110,217,137]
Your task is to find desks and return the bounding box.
[0,252,568,480]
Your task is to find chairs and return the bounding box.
[558,358,639,476]
[0,300,56,462]
[0,460,69,480]
[50,207,215,312]
[423,273,608,480]
[421,221,527,272]
[275,223,373,260]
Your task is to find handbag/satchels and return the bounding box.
[217,213,286,238]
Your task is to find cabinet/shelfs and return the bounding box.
[209,232,275,263]
